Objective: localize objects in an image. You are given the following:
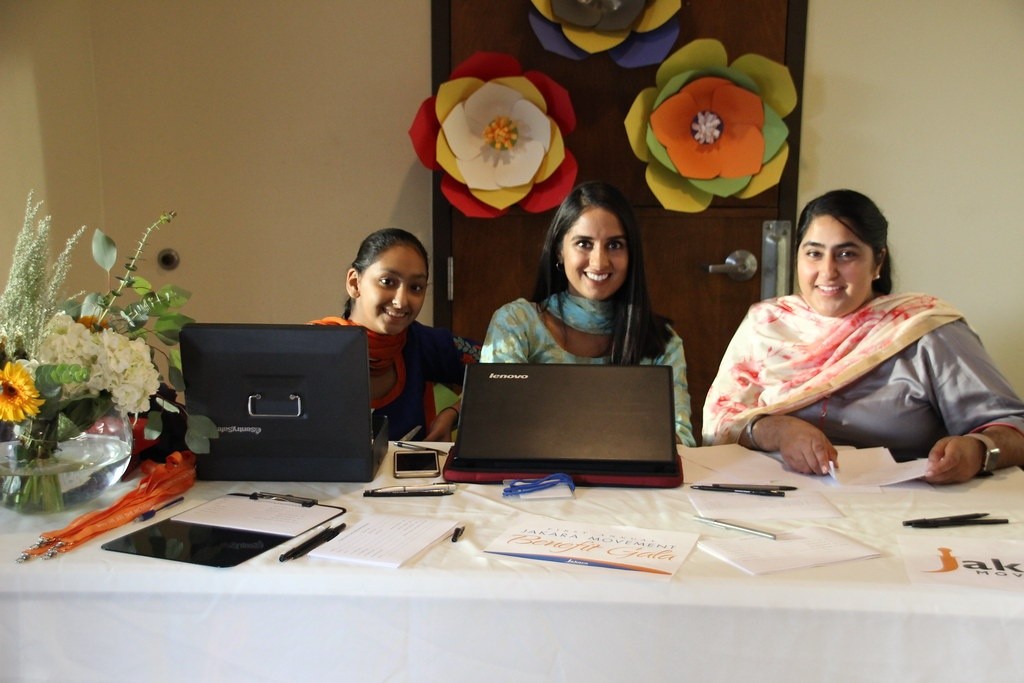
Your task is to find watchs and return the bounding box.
[963,433,1001,471]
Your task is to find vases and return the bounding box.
[1,389,131,513]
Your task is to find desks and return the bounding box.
[1,445,1024,681]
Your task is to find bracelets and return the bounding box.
[746,414,768,451]
[439,406,459,431]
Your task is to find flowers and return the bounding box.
[1,188,203,450]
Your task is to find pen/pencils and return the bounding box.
[691,482,801,497]
[690,513,778,542]
[134,494,185,523]
[900,512,1010,530]
[393,442,448,456]
[365,482,458,499]
[279,522,347,563]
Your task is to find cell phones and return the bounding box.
[393,451,440,479]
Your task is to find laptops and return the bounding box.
[449,362,680,477]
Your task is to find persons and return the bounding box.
[702,189,1024,485]
[306,227,484,442]
[481,181,698,447]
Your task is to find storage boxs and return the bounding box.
[179,323,389,484]
